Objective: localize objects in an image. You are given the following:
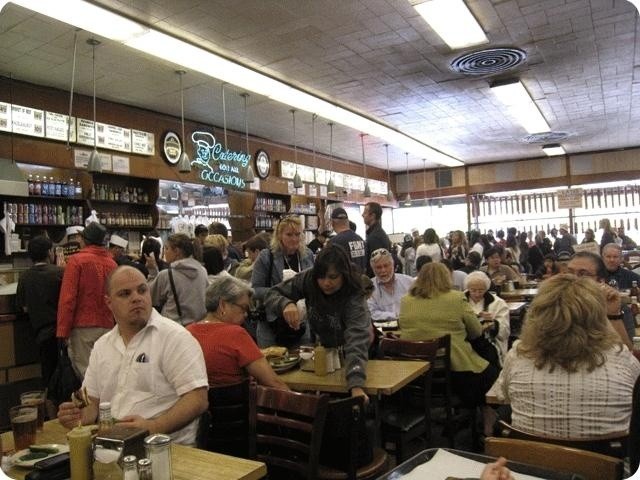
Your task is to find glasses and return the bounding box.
[232,303,250,313]
[370,250,387,256]
[567,270,598,278]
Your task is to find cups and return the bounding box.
[20,391,45,431]
[255,197,287,213]
[9,405,38,451]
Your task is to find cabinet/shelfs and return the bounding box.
[191,214,231,238]
[0,174,160,265]
[253,191,317,234]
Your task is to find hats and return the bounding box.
[556,251,571,262]
[77,221,107,246]
[66,226,84,236]
[110,235,129,249]
[560,223,568,231]
[332,207,348,219]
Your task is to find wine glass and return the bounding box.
[157,203,231,235]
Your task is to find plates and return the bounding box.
[265,354,301,373]
[10,443,69,466]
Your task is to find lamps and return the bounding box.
[541,142,567,157]
[411,0,490,50]
[490,77,552,134]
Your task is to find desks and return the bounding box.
[483,368,510,406]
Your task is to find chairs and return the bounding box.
[385,331,478,450]
[249,381,388,480]
[483,436,623,480]
[378,338,437,465]
[196,378,249,453]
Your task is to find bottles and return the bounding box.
[96,212,153,227]
[314,342,327,375]
[67,402,172,479]
[630,297,640,329]
[630,281,640,296]
[91,183,149,202]
[27,174,82,199]
[256,214,280,228]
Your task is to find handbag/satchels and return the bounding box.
[47,357,83,400]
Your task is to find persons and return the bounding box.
[14,221,289,391]
[56,265,209,446]
[251,201,511,419]
[496,217,639,447]
